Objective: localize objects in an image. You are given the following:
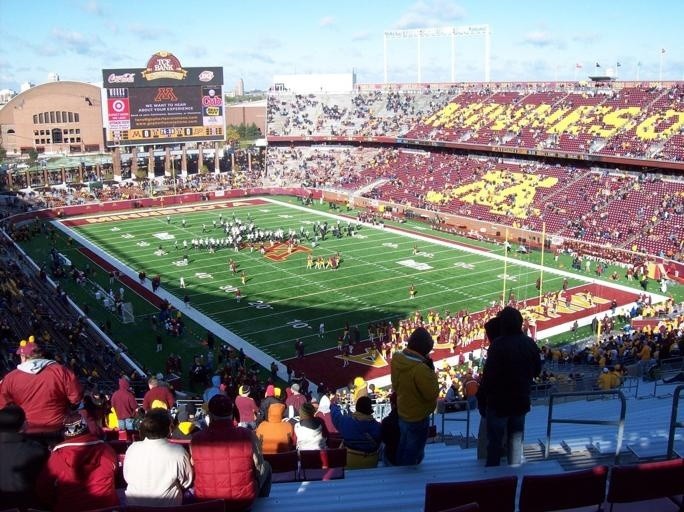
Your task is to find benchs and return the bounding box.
[1,217,153,394]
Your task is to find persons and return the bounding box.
[0,401,48,510]
[0,84,683,469]
[119,408,192,507]
[187,394,272,512]
[29,411,119,512]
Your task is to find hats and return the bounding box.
[300,402,316,419]
[209,394,233,416]
[242,385,251,394]
[356,397,374,415]
[16,342,44,358]
[62,411,89,439]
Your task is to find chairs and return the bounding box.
[260,428,349,482]
[422,456,682,512]
[266,86,684,267]
